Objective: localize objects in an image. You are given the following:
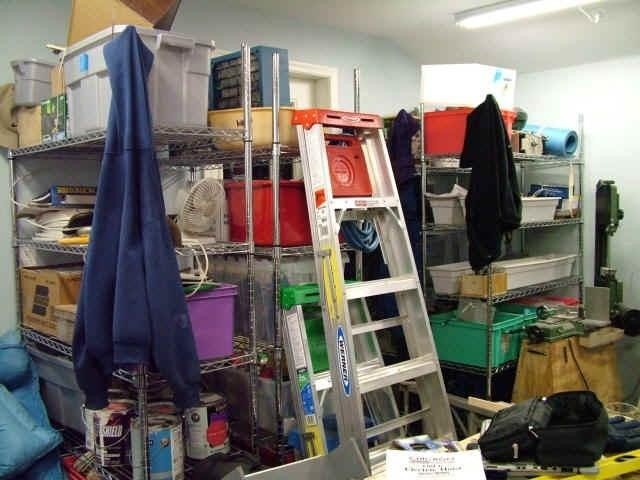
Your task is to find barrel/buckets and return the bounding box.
[79,399,134,469]
[129,415,184,480]
[185,394,230,459]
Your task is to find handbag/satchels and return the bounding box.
[480,388,607,468]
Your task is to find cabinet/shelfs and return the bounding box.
[381,103,587,401]
[8,40,256,480]
[174,53,365,466]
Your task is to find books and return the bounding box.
[472,418,599,477]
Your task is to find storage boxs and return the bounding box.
[59,25,215,138]
[420,60,518,154]
[427,292,580,373]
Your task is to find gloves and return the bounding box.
[605,415,639,453]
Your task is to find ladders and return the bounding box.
[296,108,458,456]
[277,280,410,454]
